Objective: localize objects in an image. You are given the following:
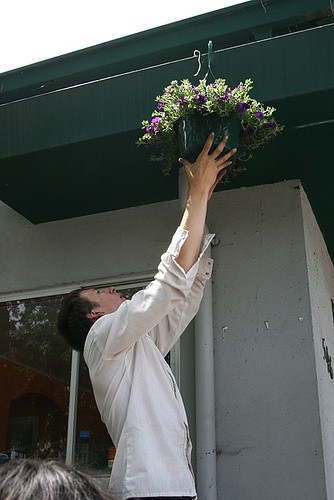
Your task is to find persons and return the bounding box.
[0,457,109,500]
[56,133,237,500]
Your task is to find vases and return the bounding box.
[172,110,243,162]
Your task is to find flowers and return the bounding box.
[138,77,283,187]
